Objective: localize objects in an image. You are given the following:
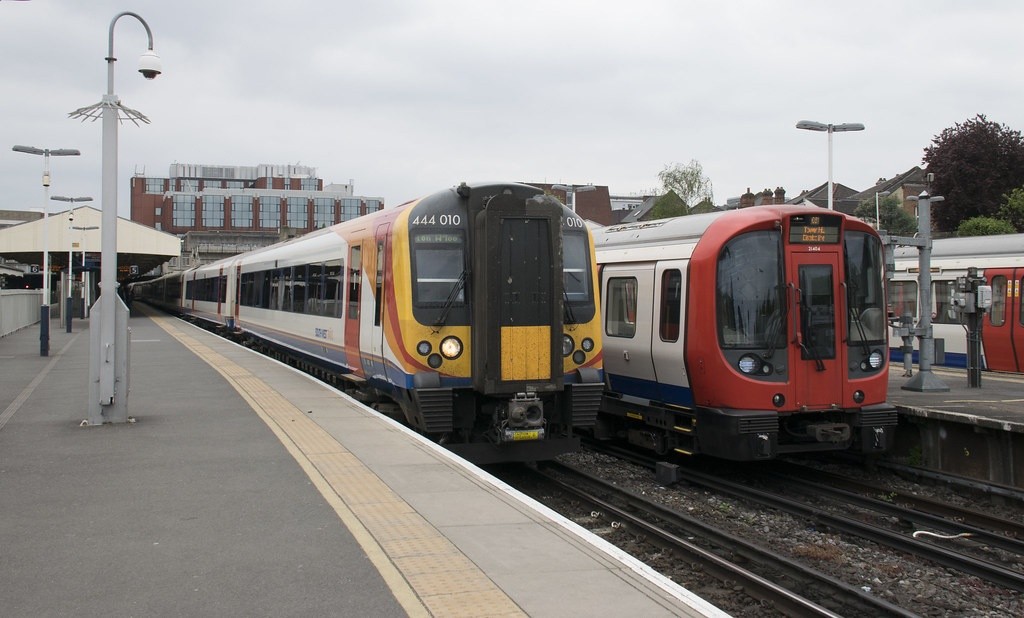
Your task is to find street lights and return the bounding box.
[12,143,81,358]
[797,121,865,209]
[70,227,101,320]
[551,184,596,214]
[875,189,891,230]
[49,193,94,332]
[88,9,163,426]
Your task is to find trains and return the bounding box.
[588,202,900,479]
[124,179,604,487]
[887,225,1024,378]
